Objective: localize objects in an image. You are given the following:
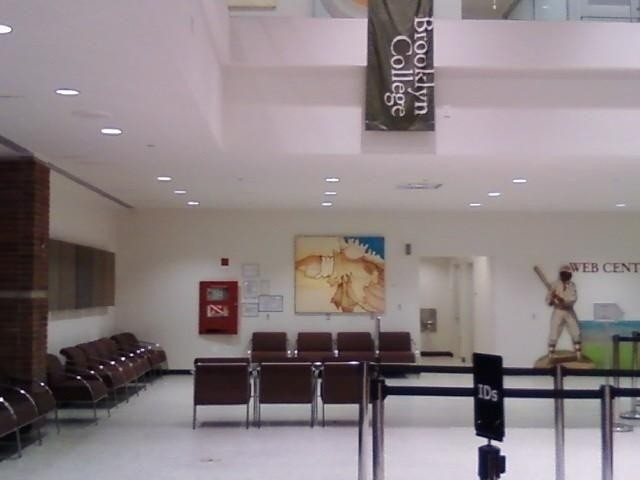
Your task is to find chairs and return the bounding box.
[0,331,165,459]
[191,331,415,431]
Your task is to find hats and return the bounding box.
[559,265,572,273]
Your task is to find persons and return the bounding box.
[544,264,583,362]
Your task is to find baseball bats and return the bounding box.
[534,266,559,299]
